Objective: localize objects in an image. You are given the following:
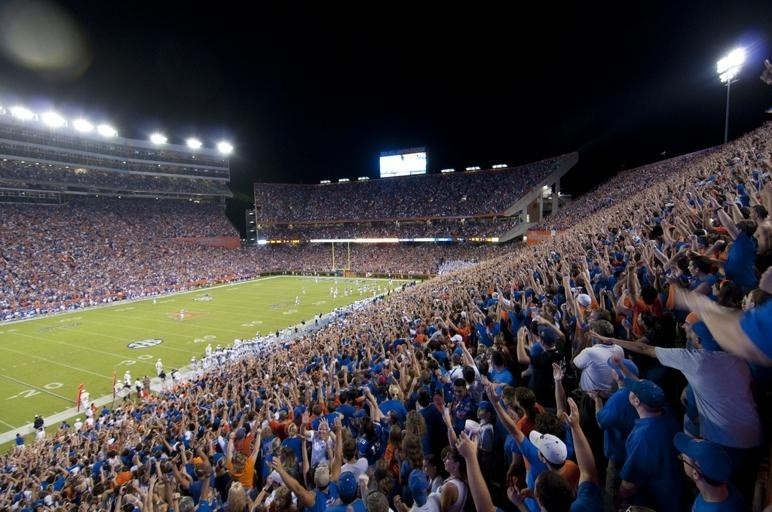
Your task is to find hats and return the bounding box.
[623,376,666,409]
[607,356,640,381]
[576,294,592,307]
[337,471,359,500]
[409,469,428,506]
[538,325,557,347]
[313,462,330,489]
[528,429,568,464]
[673,431,731,484]
[450,335,462,343]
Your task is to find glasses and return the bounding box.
[677,454,700,474]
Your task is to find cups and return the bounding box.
[464,418,480,442]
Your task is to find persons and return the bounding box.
[0,122,254,320]
[0,343,770,511]
[256,119,771,359]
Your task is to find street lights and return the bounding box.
[716,47,746,145]
